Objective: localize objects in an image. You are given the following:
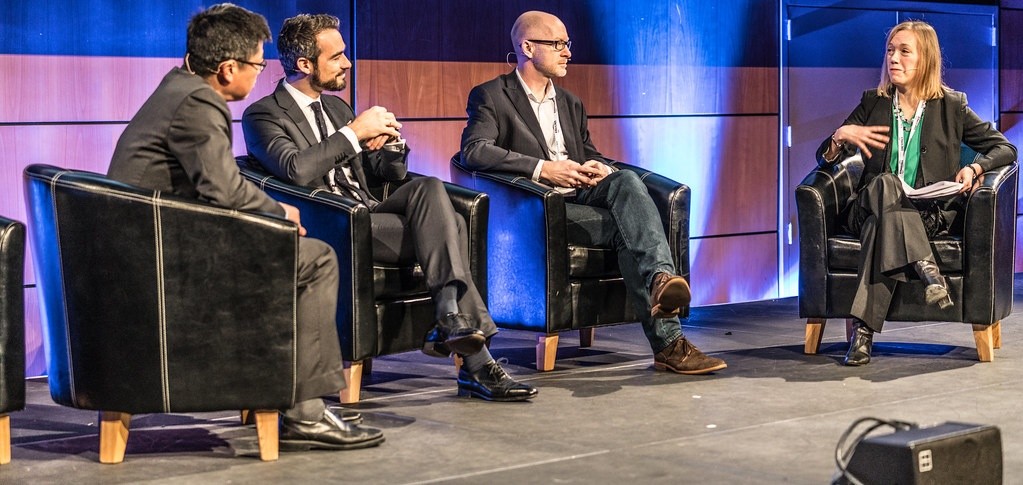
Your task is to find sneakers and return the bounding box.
[654,338,728,374]
[650,272,691,318]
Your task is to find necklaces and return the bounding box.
[893,89,927,131]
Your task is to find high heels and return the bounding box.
[844,318,874,365]
[915,259,955,310]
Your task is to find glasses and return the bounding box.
[213,55,267,72]
[519,39,572,52]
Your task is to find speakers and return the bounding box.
[833,420,1003,485]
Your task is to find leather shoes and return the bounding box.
[457,360,538,402]
[278,410,386,452]
[421,313,485,357]
[326,406,364,426]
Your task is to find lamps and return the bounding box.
[831,416,1004,485]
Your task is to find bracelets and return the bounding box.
[385,134,396,143]
[965,163,979,177]
[832,134,847,150]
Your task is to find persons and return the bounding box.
[814,19,1019,365]
[107,2,386,450]
[458,9,728,373]
[240,13,540,401]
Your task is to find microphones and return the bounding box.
[567,58,571,61]
[903,68,917,73]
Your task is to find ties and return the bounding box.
[310,101,373,213]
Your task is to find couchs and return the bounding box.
[232,154,491,403]
[0,215,26,467]
[448,148,692,371]
[22,161,299,463]
[792,152,1019,363]
